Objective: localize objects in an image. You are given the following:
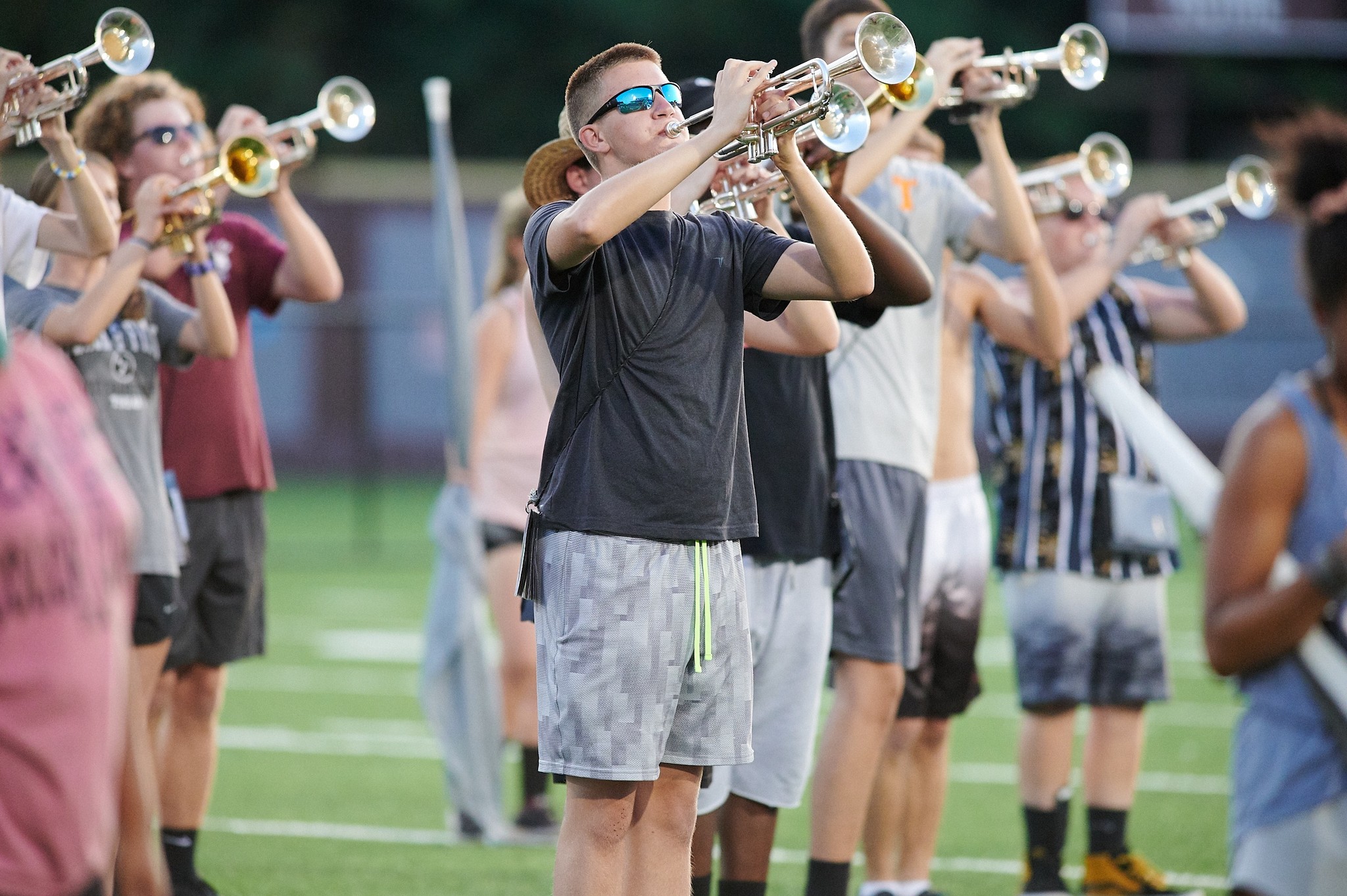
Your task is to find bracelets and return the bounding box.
[128,236,155,250]
[48,149,87,179]
[184,256,217,275]
[1309,543,1347,602]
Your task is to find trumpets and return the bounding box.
[1019,133,1133,216]
[665,13,918,164]
[932,22,1110,112]
[177,74,380,161]
[775,52,936,203]
[0,8,153,149]
[687,81,870,216]
[112,138,282,259]
[1129,153,1280,265]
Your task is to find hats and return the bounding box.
[676,77,716,133]
[523,105,585,210]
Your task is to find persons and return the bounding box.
[420,0,1075,896]
[1205,110,1347,896]
[523,39,874,894]
[0,42,344,896]
[966,155,1247,896]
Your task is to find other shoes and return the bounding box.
[1082,850,1204,896]
[514,806,562,834]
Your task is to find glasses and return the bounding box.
[1065,198,1117,224]
[130,120,201,146]
[578,82,683,143]
